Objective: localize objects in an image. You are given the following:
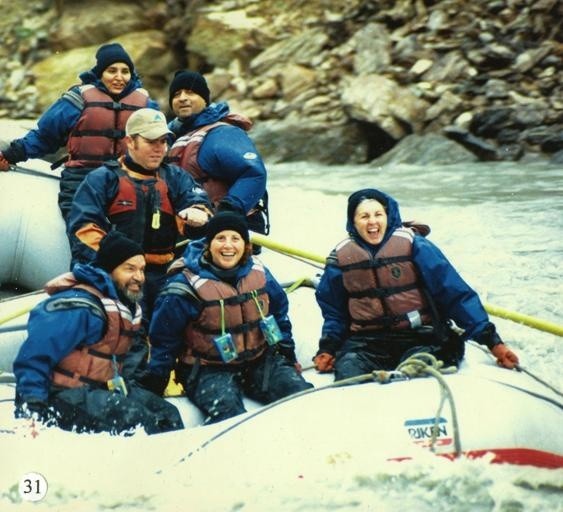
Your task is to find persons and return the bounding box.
[0,43,160,270]
[140,209,315,426]
[314,189,519,386]
[163,68,271,252]
[12,229,184,438]
[67,107,214,317]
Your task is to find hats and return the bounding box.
[168,69,210,106]
[126,109,176,142]
[207,212,249,245]
[95,231,145,271]
[96,44,135,76]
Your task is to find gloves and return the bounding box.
[178,208,205,229]
[491,342,518,369]
[0,150,9,171]
[314,353,335,372]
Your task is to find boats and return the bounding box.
[0,116,74,285]
[2,288,563,475]
